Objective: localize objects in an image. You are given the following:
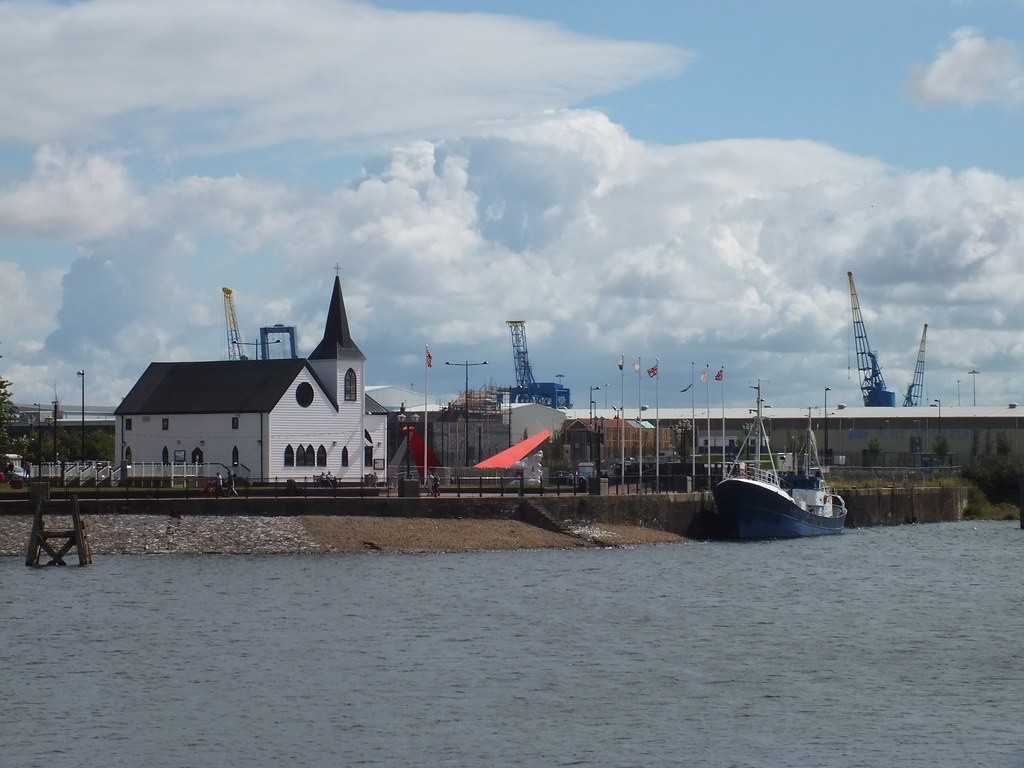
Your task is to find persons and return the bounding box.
[214,472,224,498]
[8,462,14,474]
[24,463,30,477]
[815,469,821,486]
[603,471,608,477]
[425,471,440,497]
[225,470,239,497]
[366,472,378,486]
[320,470,334,488]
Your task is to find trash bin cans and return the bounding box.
[679,476,692,492]
[398,476,421,496]
[588,474,609,495]
[30,482,50,499]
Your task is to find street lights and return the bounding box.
[396,412,424,497]
[444,361,490,467]
[955,379,963,406]
[589,385,601,427]
[52,400,60,465]
[824,387,832,466]
[76,369,86,464]
[967,370,982,408]
[934,399,942,436]
[555,374,565,385]
[34,402,42,479]
[613,405,624,458]
[231,340,283,360]
[604,383,612,410]
[632,355,650,487]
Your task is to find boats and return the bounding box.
[710,378,849,542]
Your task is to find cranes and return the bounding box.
[506,320,541,403]
[847,271,928,409]
[222,286,252,359]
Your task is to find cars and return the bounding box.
[7,466,29,481]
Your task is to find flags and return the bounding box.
[617,359,623,370]
[647,365,657,377]
[715,369,723,381]
[700,367,708,382]
[427,350,432,367]
[680,383,692,392]
[633,361,639,372]
